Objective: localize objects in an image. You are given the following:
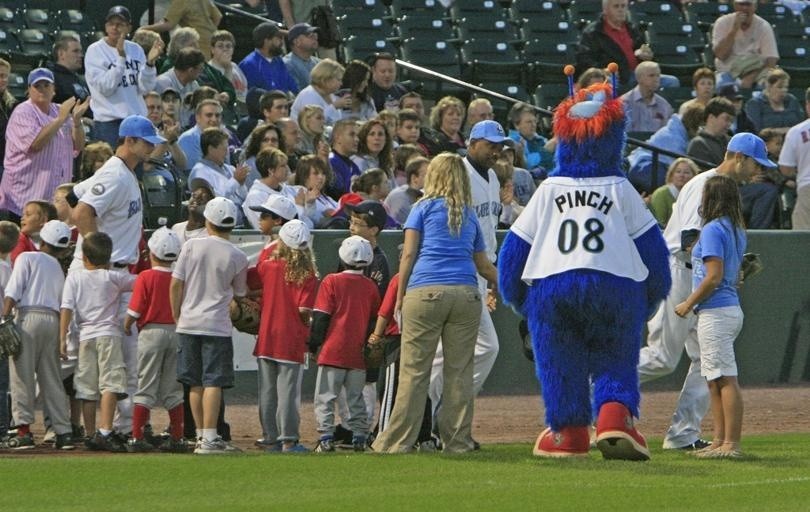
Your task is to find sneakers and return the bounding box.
[1,424,235,453]
[662,434,715,452]
[686,442,744,459]
[255,425,479,452]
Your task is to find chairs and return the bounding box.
[0,7,106,100]
[144,158,182,230]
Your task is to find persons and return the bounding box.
[1,0,810,462]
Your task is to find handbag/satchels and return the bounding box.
[310,5,344,49]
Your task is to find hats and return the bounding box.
[716,81,749,103]
[247,194,299,223]
[118,112,169,150]
[726,130,780,171]
[202,196,239,231]
[25,69,60,87]
[469,119,513,145]
[189,177,220,203]
[251,18,289,47]
[103,5,132,27]
[335,236,377,267]
[344,196,389,230]
[36,219,72,249]
[159,86,182,98]
[285,22,321,42]
[147,225,184,263]
[269,217,315,251]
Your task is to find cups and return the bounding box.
[383,97,400,113]
[340,88,353,112]
